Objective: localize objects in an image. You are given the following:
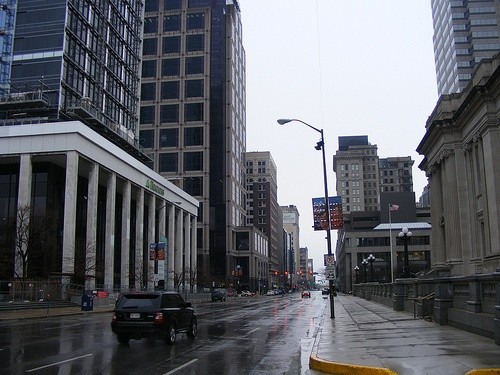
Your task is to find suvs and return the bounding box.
[108,291,199,344]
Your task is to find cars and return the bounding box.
[212,289,256,301]
[267,288,285,296]
[301,289,311,299]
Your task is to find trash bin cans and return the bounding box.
[81,294,95,312]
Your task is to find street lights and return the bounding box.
[354,266,360,285]
[367,254,375,282]
[276,118,336,320]
[154,200,183,289]
[362,259,369,283]
[399,228,414,279]
